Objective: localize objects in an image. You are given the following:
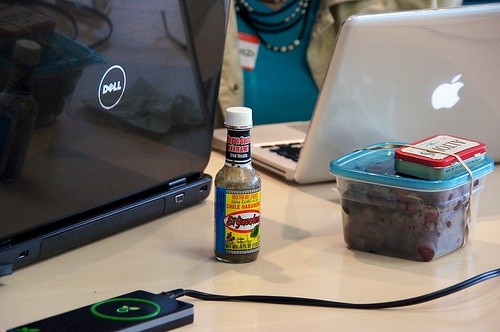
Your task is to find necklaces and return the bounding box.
[236,1,312,52]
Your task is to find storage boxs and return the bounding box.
[393,135,486,180]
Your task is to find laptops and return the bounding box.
[212,2,500,185]
[0,0,232,278]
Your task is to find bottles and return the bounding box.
[214,106,262,264]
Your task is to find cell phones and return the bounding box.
[6,290,195,332]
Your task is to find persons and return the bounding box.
[214,0,462,128]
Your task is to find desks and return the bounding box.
[0,121,500,332]
